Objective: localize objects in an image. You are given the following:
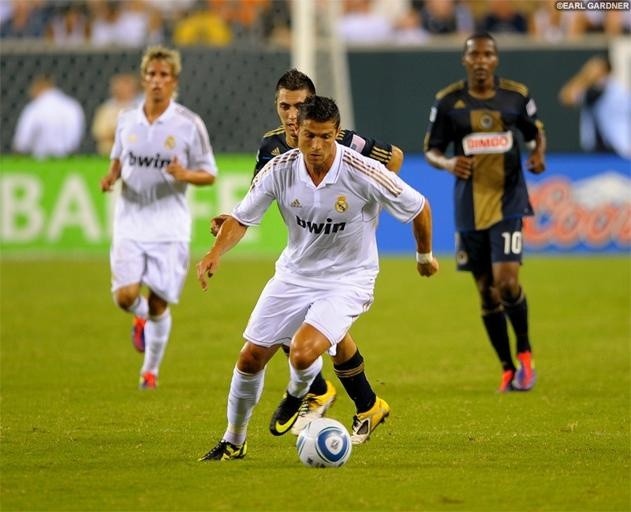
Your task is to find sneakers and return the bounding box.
[512,352,537,390]
[270,391,305,436]
[139,372,154,388]
[199,439,247,460]
[350,396,391,444]
[291,379,336,435]
[132,316,146,351]
[496,369,514,392]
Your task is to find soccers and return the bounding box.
[296,418,352,468]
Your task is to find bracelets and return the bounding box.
[416,248,434,266]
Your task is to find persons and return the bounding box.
[1,1,630,157]
[98,45,219,390]
[209,68,405,447]
[195,94,441,464]
[421,32,549,393]
